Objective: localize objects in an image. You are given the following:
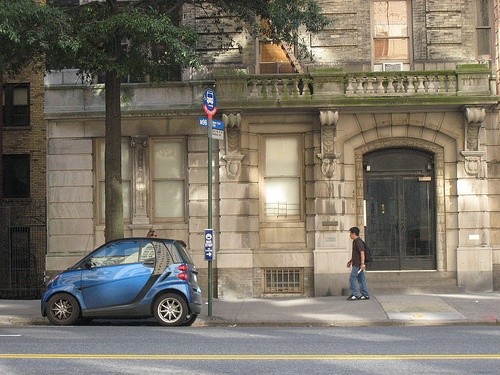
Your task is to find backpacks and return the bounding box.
[364,242,373,264]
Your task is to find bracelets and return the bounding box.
[361,262,365,265]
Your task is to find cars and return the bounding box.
[41,237,203,326]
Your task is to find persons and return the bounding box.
[146,231,159,238]
[346,226,370,301]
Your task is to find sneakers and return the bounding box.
[357,295,369,300]
[348,293,357,300]
